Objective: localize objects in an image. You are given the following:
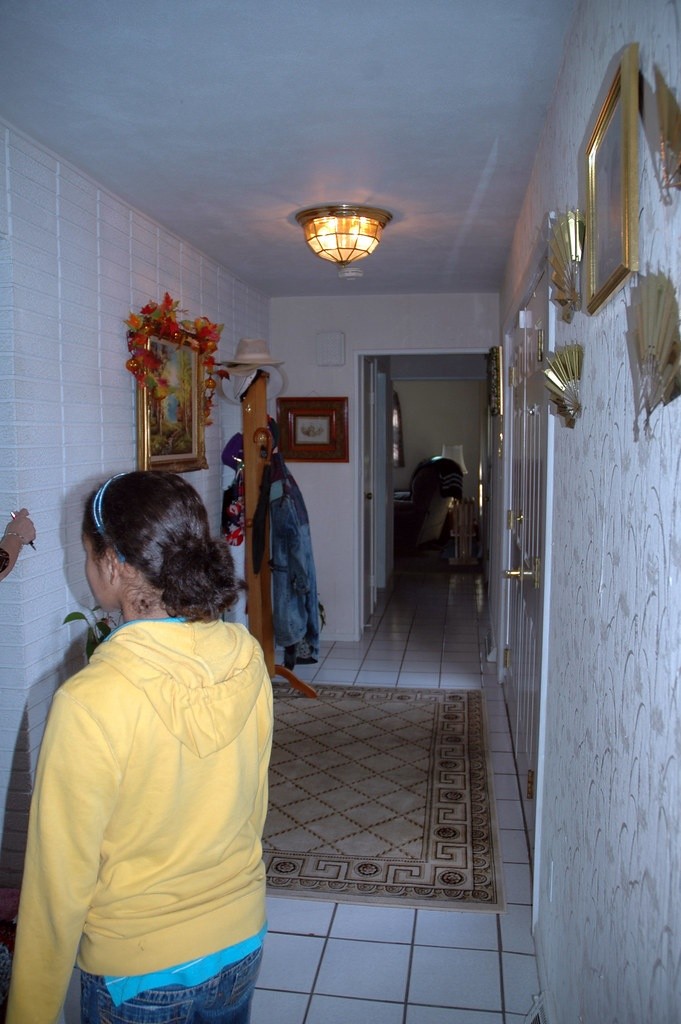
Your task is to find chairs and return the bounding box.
[392,455,463,569]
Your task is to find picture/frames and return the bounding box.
[487,346,503,416]
[262,681,506,914]
[584,42,641,318]
[137,316,209,472]
[275,396,350,463]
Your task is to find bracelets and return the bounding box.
[4,531,24,553]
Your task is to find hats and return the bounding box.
[221,366,282,404]
[223,338,284,365]
[221,432,248,480]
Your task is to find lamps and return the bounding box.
[295,205,393,271]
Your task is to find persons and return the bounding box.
[7,473,273,1022]
[0,509,36,581]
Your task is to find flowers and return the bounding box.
[122,289,225,426]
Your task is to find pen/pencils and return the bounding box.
[10,512,37,551]
